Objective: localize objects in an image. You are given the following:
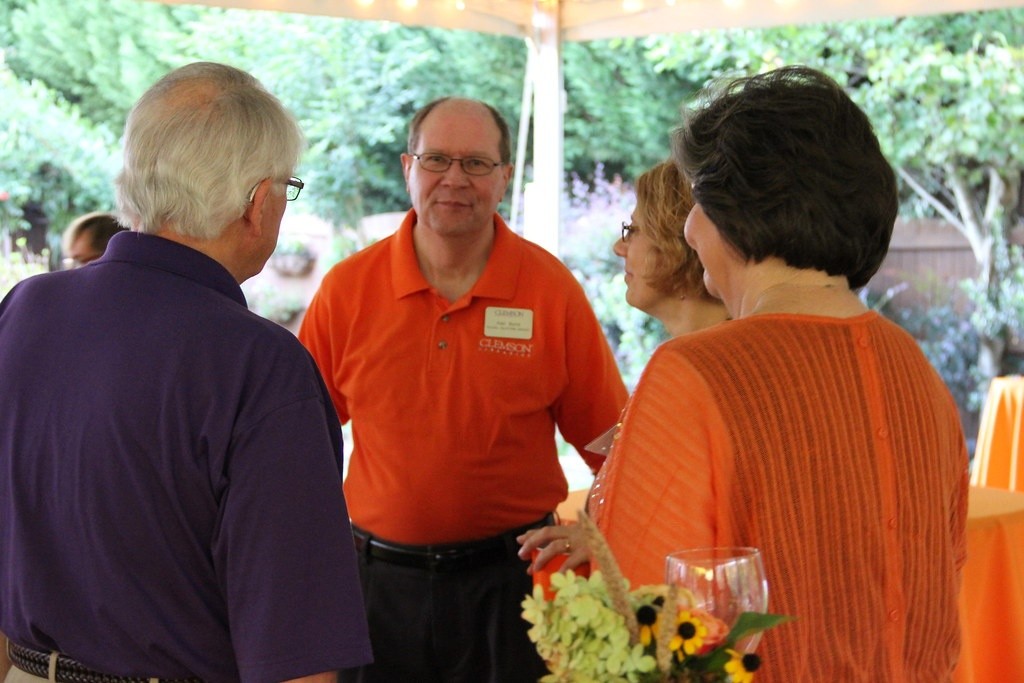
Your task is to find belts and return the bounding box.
[349,511,554,569]
[8,641,200,683]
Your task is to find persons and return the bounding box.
[0,61,374,683]
[298,96,632,683]
[588,64,970,682]
[515,157,733,592]
[61,210,130,270]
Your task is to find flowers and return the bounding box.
[520,509,800,683]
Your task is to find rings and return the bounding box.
[564,538,572,554]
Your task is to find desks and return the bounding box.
[953,485,1024,683]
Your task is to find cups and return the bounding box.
[531,546,590,601]
[664,546,768,683]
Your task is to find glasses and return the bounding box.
[622,222,641,242]
[410,152,503,176]
[249,176,304,202]
